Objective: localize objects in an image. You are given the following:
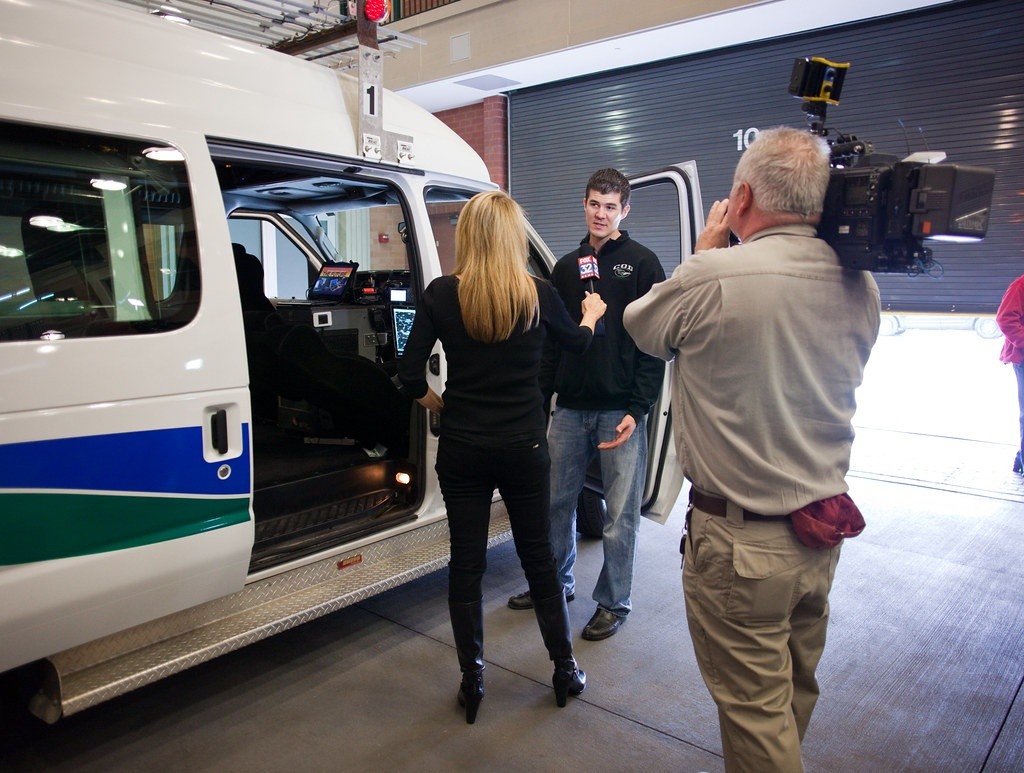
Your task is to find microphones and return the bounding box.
[577,243,600,294]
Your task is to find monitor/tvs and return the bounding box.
[307,262,359,303]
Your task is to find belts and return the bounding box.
[688,485,791,521]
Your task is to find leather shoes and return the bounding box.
[582,608,627,641]
[508,591,575,609]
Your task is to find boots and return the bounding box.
[448,594,486,724]
[532,585,587,707]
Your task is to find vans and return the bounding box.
[0,0,709,728]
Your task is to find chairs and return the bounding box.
[229,243,401,446]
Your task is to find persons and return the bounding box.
[509,170,666,639]
[996,276,1024,476]
[396,190,607,723]
[622,127,881,773]
